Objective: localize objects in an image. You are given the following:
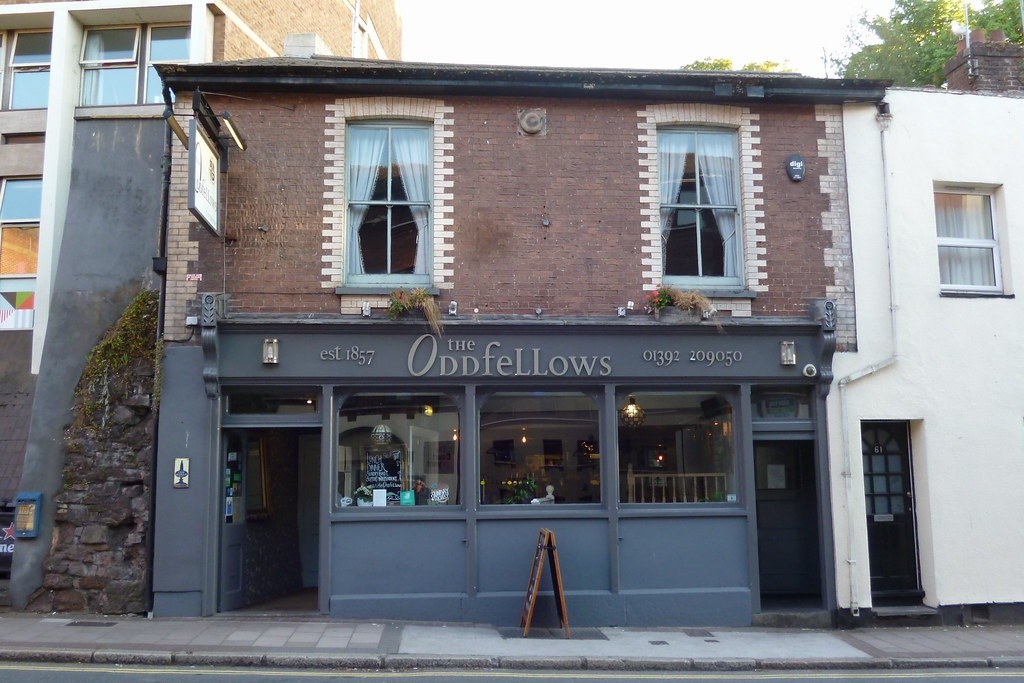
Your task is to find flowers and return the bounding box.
[352,486,373,501]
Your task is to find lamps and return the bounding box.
[262,338,278,364]
[619,398,645,429]
[370,424,392,448]
[421,404,434,417]
[162,109,248,151]
[780,340,796,365]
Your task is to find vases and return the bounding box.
[356,497,372,507]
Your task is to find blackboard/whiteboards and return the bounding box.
[520,528,549,637]
